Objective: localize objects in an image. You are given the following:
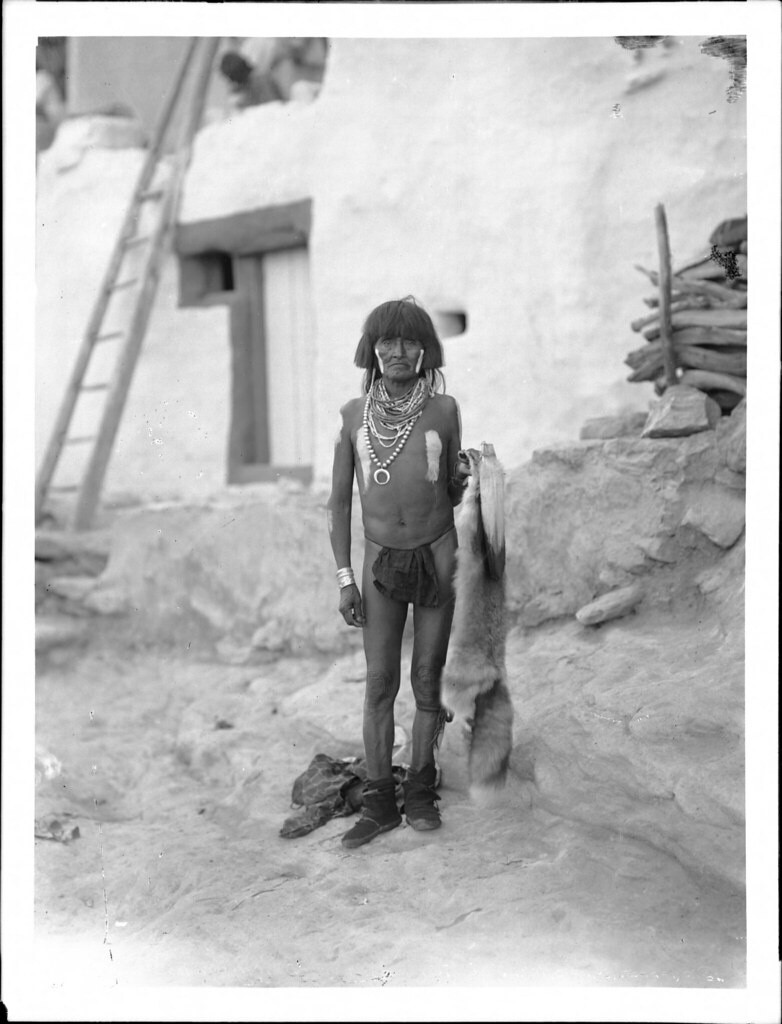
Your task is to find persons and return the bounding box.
[326,299,481,849]
[221,52,280,108]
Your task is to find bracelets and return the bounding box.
[336,567,356,589]
[451,462,469,490]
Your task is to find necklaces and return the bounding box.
[363,378,430,485]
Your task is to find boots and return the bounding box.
[341,776,402,848]
[401,764,442,829]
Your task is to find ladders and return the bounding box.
[34,37,220,532]
